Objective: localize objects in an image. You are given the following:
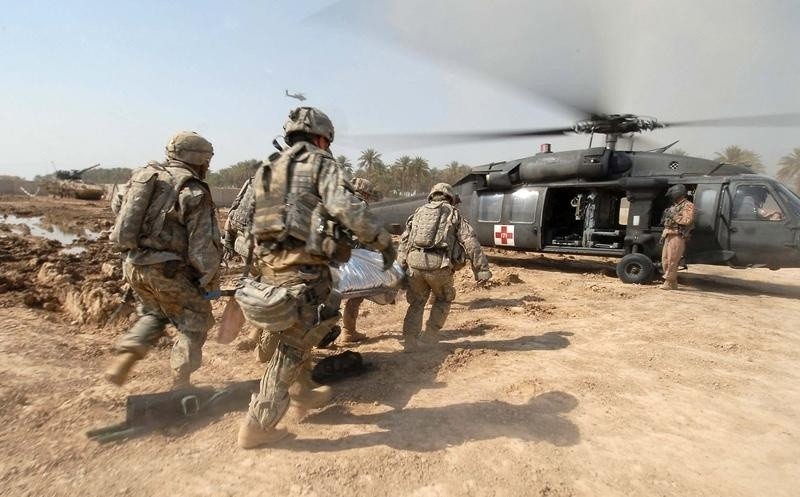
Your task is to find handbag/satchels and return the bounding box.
[305,201,351,263]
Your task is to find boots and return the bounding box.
[173,376,214,399]
[658,280,678,290]
[290,385,333,414]
[238,423,288,448]
[105,352,138,386]
[341,328,366,342]
[404,327,438,353]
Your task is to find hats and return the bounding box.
[351,178,382,201]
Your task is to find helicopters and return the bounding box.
[309,0,800,285]
[284,89,307,102]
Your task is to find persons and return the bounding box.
[315,177,373,348]
[223,106,396,449]
[394,181,493,354]
[659,184,697,290]
[103,131,224,402]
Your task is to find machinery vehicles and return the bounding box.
[55,164,105,200]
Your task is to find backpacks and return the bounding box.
[413,201,444,247]
[109,168,158,249]
[234,278,298,331]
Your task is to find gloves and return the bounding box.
[381,246,397,272]
[199,285,220,300]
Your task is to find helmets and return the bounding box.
[427,182,456,205]
[665,184,686,202]
[168,132,213,166]
[283,106,334,142]
